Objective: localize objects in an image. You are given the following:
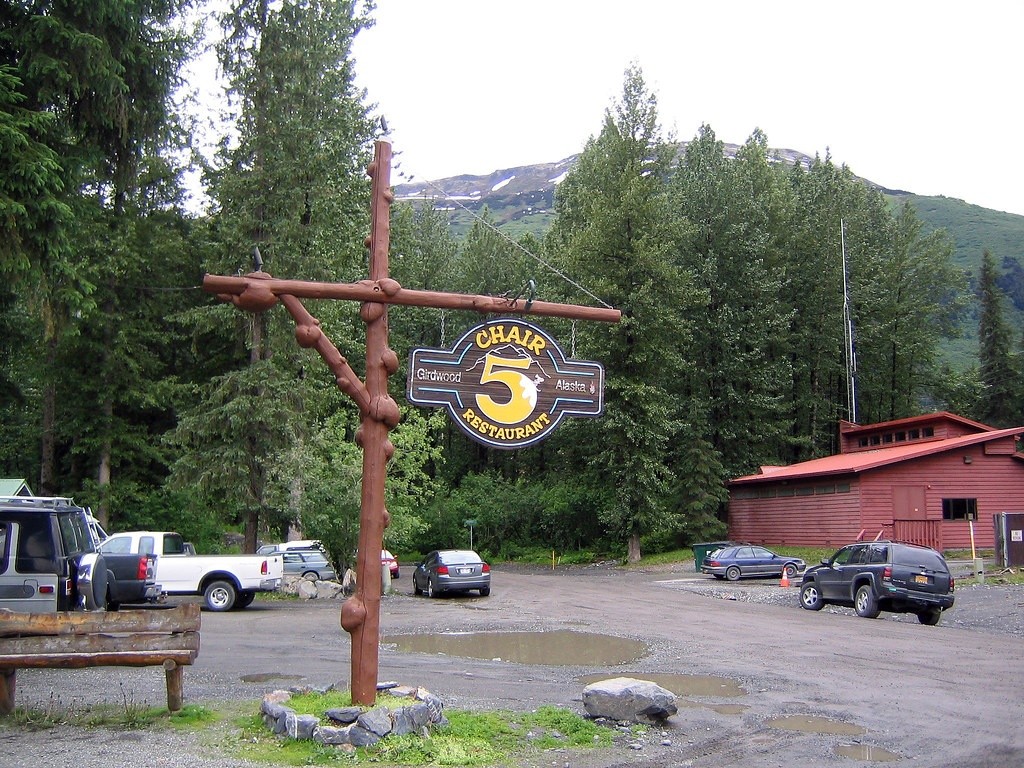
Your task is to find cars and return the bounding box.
[412,549,491,596]
[267,549,337,581]
[381,549,400,578]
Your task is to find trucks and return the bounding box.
[95,530,285,611]
[257,540,327,558]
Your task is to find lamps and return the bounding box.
[963,455,972,463]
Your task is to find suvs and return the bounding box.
[0,495,108,614]
[798,540,956,625]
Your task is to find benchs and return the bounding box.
[0,602,201,715]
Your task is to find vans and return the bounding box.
[700,541,808,581]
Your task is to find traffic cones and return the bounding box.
[780,567,790,587]
